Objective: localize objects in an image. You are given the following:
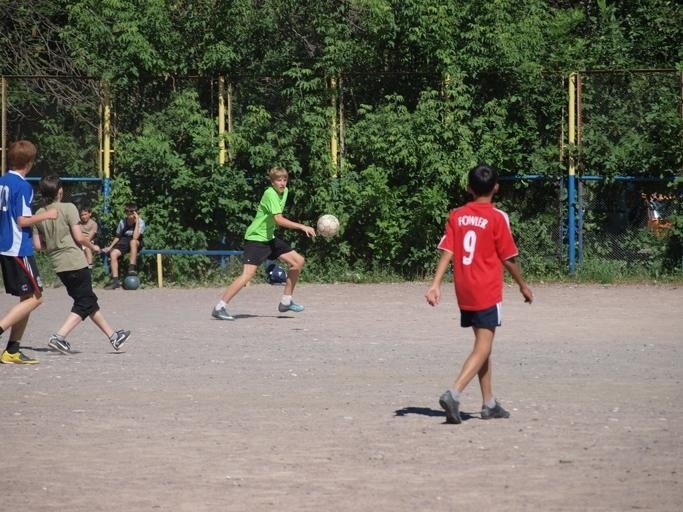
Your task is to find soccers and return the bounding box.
[124,275,141,290]
[316,214,340,236]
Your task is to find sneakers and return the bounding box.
[105,280,119,288]
[127,264,138,276]
[482,401,509,418]
[278,301,304,312]
[110,330,131,350]
[48,335,71,354]
[212,307,234,319]
[439,390,461,423]
[0,350,38,364]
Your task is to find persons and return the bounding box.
[30,173,131,356]
[211,166,317,321]
[77,205,98,273]
[101,202,145,290]
[423,165,534,425]
[0,140,59,365]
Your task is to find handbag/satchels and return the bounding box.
[268,264,286,282]
